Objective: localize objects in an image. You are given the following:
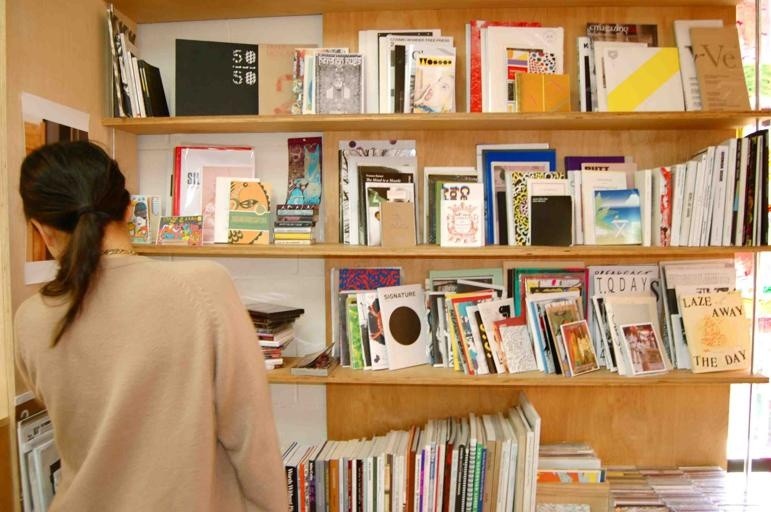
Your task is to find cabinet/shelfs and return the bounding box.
[103,2,770,469]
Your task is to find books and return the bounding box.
[338,140,420,249]
[294,343,338,377]
[576,22,684,112]
[672,19,753,113]
[423,258,751,376]
[478,142,574,248]
[291,48,362,115]
[466,19,571,113]
[15,390,60,510]
[245,304,304,371]
[105,2,169,118]
[536,444,721,510]
[279,391,542,511]
[174,39,258,116]
[423,166,482,247]
[358,28,456,114]
[564,155,641,247]
[331,266,431,373]
[120,137,319,246]
[636,129,767,249]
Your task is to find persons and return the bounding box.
[15,141,289,511]
